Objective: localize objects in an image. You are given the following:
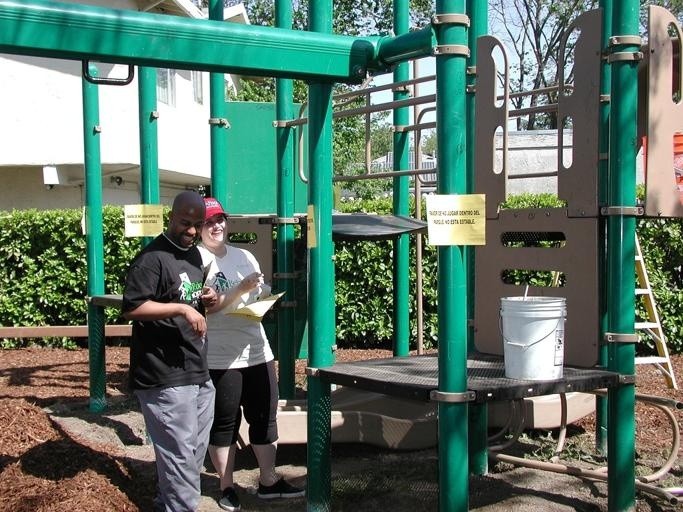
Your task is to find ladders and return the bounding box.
[633,231,678,389]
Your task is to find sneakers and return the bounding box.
[218,487,243,512]
[257,476,306,500]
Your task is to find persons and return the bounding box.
[122,188,219,512]
[191,195,305,512]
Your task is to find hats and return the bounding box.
[203,196,229,222]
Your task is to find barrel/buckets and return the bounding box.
[498,295,568,382]
